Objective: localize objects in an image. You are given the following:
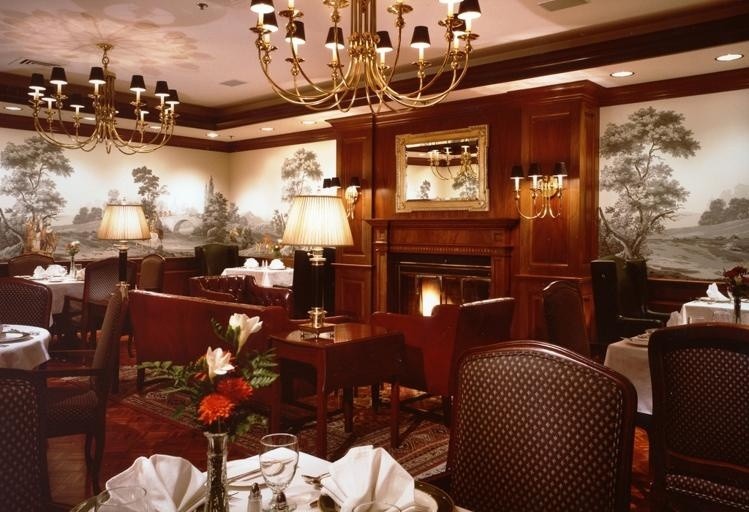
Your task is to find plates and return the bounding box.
[218,464,266,487]
[620,297,749,348]
[16,275,86,284]
[318,477,457,512]
[0,331,31,343]
[72,489,205,511]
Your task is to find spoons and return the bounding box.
[301,471,330,484]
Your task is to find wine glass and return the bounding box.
[258,433,300,511]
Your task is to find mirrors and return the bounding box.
[394,124,490,214]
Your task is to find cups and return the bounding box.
[95,485,152,512]
[350,502,401,512]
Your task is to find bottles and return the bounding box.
[246,481,264,511]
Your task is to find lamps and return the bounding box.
[27,42,181,156]
[280,194,355,341]
[250,0,483,115]
[509,161,569,220]
[322,175,361,220]
[97,203,152,302]
[426,138,479,181]
